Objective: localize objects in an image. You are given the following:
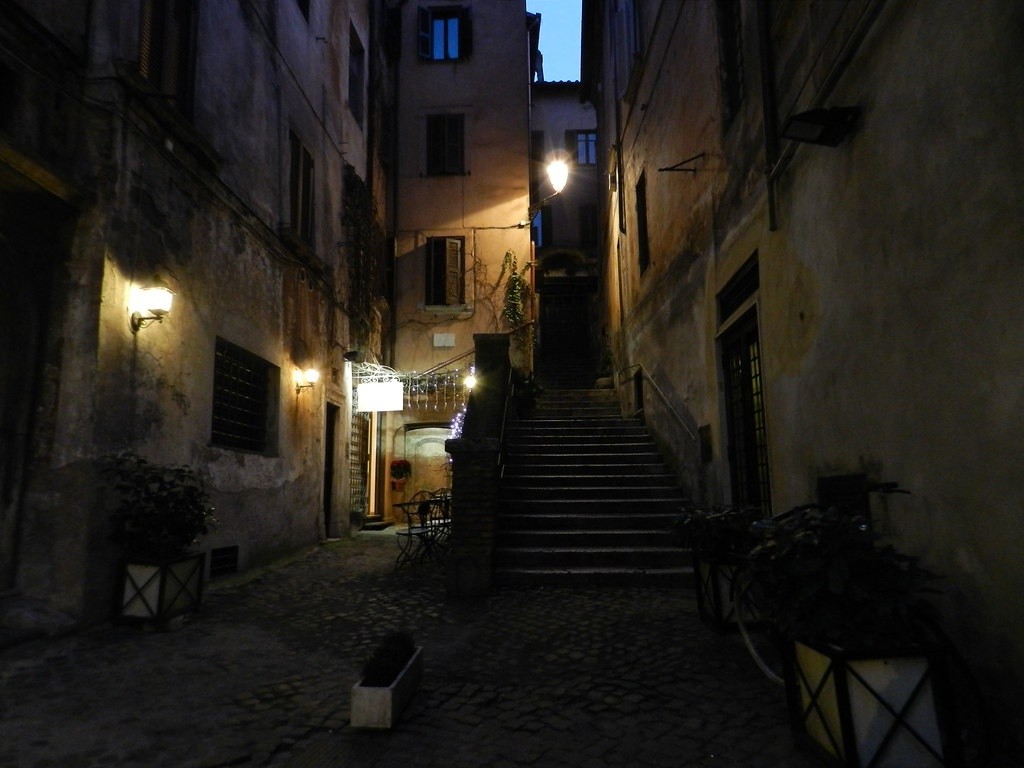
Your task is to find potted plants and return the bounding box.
[666,490,981,768]
[349,627,424,729]
[108,460,214,620]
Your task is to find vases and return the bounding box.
[393,476,406,491]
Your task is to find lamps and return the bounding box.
[780,106,862,152]
[296,367,323,395]
[126,276,176,334]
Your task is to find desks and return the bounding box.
[392,496,451,563]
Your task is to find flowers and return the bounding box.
[391,459,412,478]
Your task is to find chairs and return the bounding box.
[395,487,451,576]
